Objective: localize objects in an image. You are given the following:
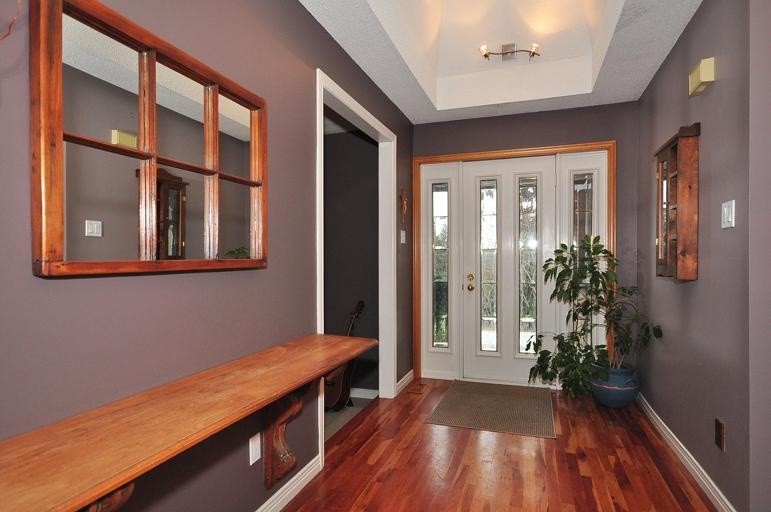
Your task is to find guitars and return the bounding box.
[323,301,365,412]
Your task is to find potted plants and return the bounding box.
[525,235,662,410]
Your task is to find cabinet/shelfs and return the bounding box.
[653,120,700,282]
[134,168,190,261]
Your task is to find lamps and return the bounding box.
[478,41,541,60]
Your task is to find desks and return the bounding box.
[1,331,380,512]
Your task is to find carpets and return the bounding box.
[423,378,558,440]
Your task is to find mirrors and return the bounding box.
[27,0,269,281]
